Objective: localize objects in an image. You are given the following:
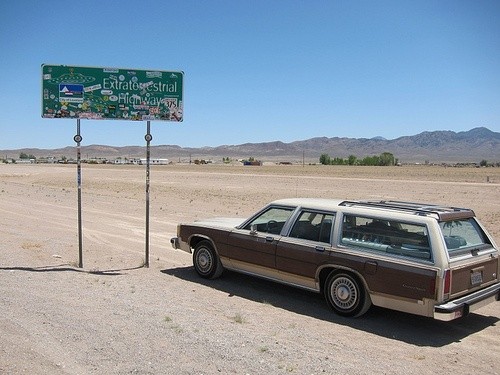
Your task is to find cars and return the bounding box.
[170,198,500,322]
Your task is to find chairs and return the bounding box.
[291,221,331,244]
